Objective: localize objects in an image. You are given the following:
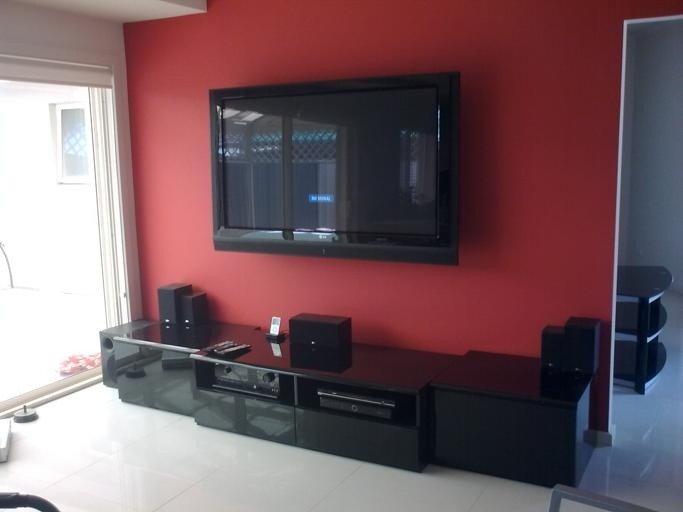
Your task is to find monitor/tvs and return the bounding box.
[208,70,459,267]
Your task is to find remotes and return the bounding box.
[206,341,231,352]
[214,342,238,354]
[220,344,251,354]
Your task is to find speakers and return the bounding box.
[541,325,563,399]
[563,316,601,396]
[157,282,192,327]
[289,313,352,373]
[181,292,206,335]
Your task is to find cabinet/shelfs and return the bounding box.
[188,331,463,479]
[614,263,672,395]
[108,318,260,418]
[428,345,599,488]
